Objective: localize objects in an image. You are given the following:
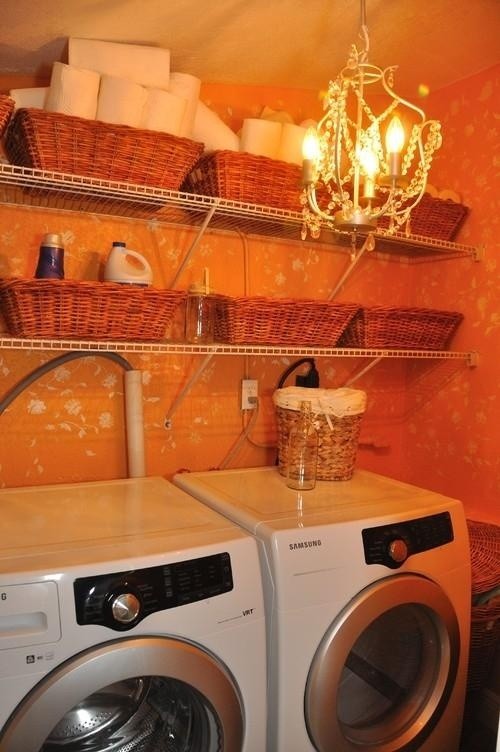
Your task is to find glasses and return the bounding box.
[298,1,444,262]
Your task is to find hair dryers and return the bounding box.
[2,473,270,749]
[177,457,475,752]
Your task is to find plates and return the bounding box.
[0,158,482,361]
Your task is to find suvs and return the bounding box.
[104,240,154,286]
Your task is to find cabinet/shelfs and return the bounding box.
[35,232,68,279]
[283,398,321,489]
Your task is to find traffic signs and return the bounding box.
[237,375,261,417]
